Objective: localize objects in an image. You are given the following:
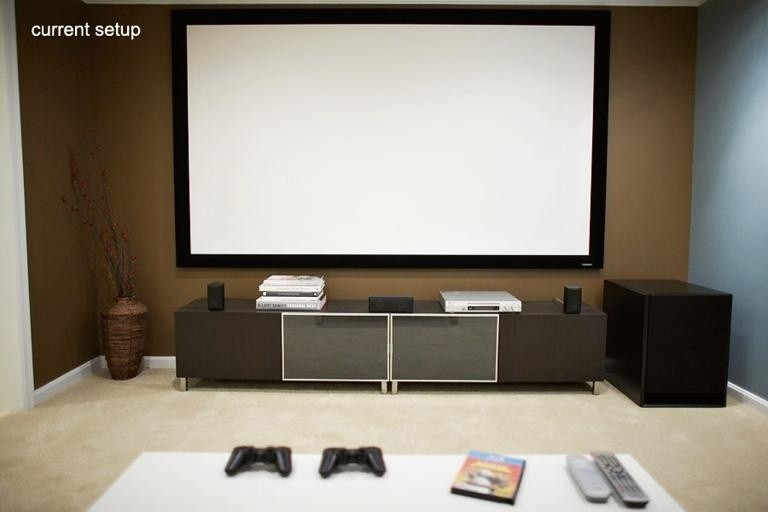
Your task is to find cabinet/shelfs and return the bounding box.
[177,298,605,393]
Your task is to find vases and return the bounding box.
[101,298,149,379]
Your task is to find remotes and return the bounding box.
[591,451,649,507]
[566,453,611,502]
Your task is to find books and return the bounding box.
[450,448,527,506]
[255,275,327,311]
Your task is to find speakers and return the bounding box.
[563,285,582,313]
[368,296,414,313]
[207,282,224,311]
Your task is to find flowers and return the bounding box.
[54,129,141,298]
